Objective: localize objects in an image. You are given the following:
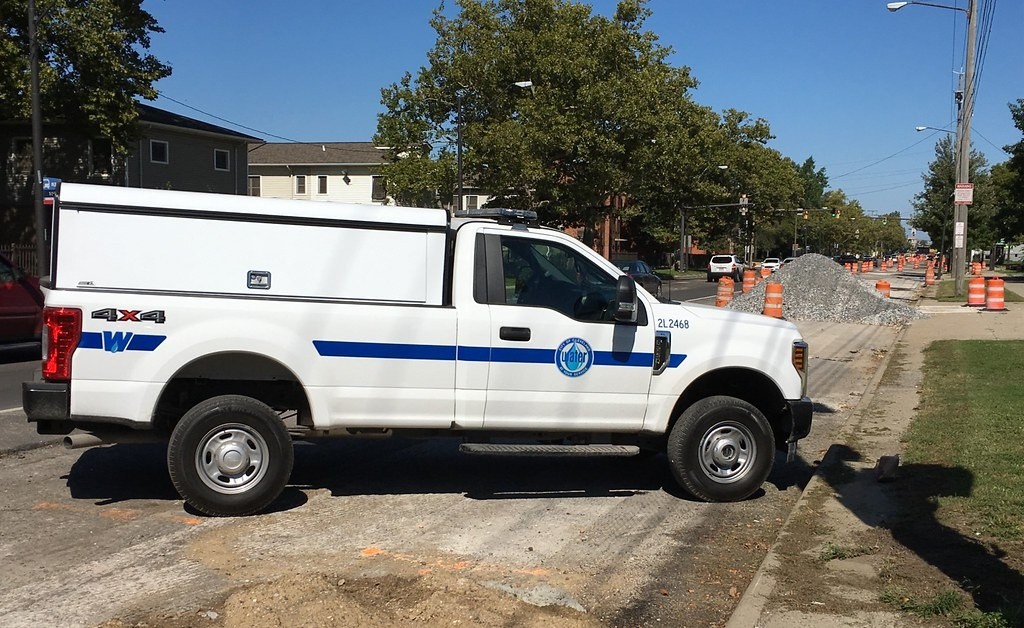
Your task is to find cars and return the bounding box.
[761,258,780,273]
[863,257,878,267]
[779,256,797,270]
[891,257,898,263]
[0,256,49,344]
[926,255,933,260]
[883,255,893,262]
[832,255,858,267]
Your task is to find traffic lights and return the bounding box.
[883,217,887,226]
[912,229,916,237]
[832,208,836,217]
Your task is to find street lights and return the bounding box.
[915,125,962,278]
[455,77,529,209]
[886,0,975,297]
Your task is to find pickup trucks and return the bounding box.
[20,177,815,506]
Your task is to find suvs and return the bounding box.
[707,253,746,282]
